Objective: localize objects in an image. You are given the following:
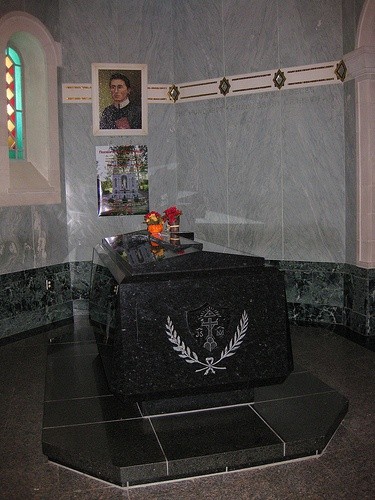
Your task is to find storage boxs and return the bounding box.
[90,230,294,406]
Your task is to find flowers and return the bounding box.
[161,205,183,226]
[148,247,164,257]
[176,249,185,255]
[142,210,163,225]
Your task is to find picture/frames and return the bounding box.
[90,61,149,137]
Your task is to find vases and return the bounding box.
[168,218,180,232]
[169,232,180,245]
[147,224,164,233]
[150,233,164,246]
